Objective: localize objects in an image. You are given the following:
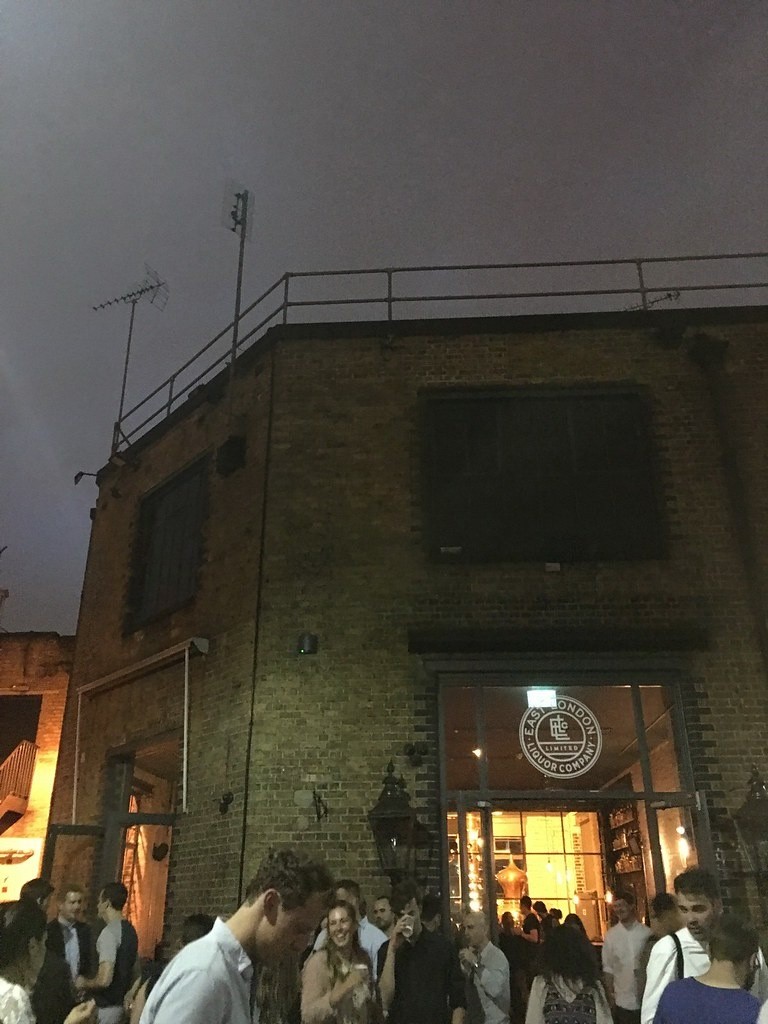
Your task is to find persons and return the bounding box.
[637,893,686,1005]
[499,896,590,1024]
[75,882,139,1023]
[652,914,764,1024]
[640,865,767,1024]
[123,913,214,1023]
[31,885,92,1023]
[601,892,654,1024]
[377,879,467,1023]
[304,879,389,984]
[1,898,99,1023]
[372,897,397,938]
[302,900,374,1023]
[139,849,335,1024]
[447,912,510,1023]
[19,878,55,912]
[417,894,442,932]
[525,924,614,1024]
